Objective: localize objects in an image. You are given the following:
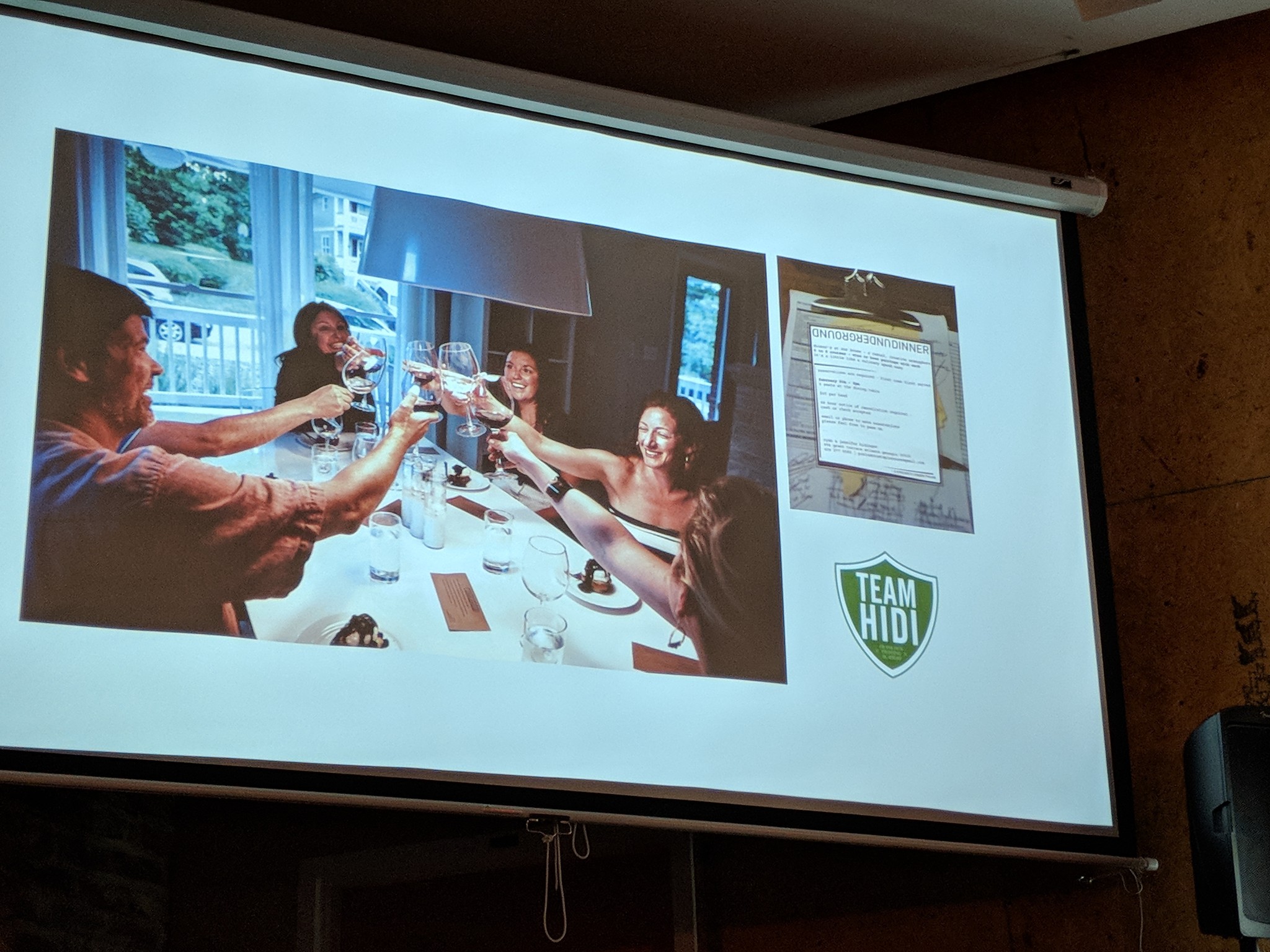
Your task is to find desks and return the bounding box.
[199,430,704,676]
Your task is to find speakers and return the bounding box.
[1181,705,1270,943]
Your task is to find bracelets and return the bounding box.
[545,476,575,502]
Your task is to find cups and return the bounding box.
[350,432,380,462]
[368,511,403,584]
[354,421,378,436]
[481,509,514,575]
[518,605,568,664]
[401,452,447,549]
[311,443,339,483]
[405,339,438,369]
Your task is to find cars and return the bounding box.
[132,284,213,343]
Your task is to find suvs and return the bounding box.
[124,258,171,301]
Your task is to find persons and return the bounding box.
[115,383,354,458]
[401,342,581,519]
[275,300,380,438]
[486,429,788,684]
[20,265,439,635]
[434,371,707,562]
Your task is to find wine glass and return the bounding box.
[519,535,570,606]
[400,364,444,454]
[350,334,387,413]
[438,341,487,439]
[321,350,385,428]
[311,414,343,450]
[468,376,519,481]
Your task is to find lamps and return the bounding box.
[357,186,593,317]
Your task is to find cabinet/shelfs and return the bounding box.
[445,293,577,474]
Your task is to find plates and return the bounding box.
[293,611,405,651]
[296,431,356,451]
[446,473,490,491]
[566,561,641,609]
